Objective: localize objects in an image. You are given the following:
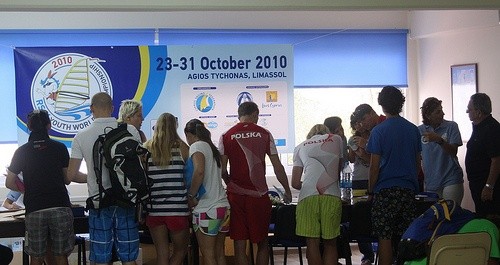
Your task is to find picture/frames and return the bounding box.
[450,63,477,142]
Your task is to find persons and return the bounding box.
[145,113,193,265]
[216,102,293,265]
[8,109,76,265]
[68,91,146,265]
[182,119,233,265]
[115,98,147,145]
[292,87,465,265]
[3,167,25,211]
[464,93,500,232]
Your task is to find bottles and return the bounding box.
[340,161,353,201]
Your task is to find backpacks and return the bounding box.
[398,198,487,261]
[84,122,155,222]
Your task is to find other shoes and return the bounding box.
[361,259,372,265]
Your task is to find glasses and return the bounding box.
[350,104,373,124]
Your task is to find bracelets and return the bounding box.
[186,192,193,200]
[436,139,444,145]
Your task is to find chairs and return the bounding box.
[23,194,492,265]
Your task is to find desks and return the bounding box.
[0,198,436,265]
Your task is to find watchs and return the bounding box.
[485,184,494,190]
